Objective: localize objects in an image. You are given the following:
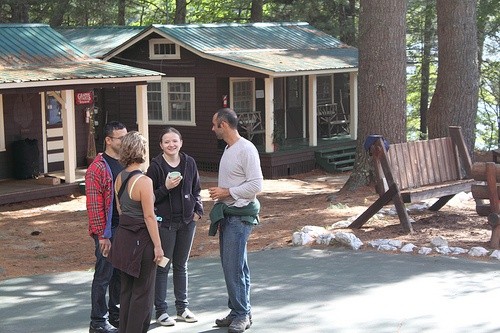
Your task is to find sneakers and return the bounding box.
[215,310,252,326]
[156,313,176,325]
[177,308,198,323]
[228,314,250,333]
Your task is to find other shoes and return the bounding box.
[108,315,120,327]
[89,322,119,333]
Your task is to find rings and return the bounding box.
[158,259,161,260]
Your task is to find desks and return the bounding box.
[236,110,265,143]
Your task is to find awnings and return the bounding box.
[206,47,360,77]
[0,60,166,90]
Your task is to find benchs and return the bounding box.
[470,160,500,249]
[348,126,488,233]
[318,103,350,137]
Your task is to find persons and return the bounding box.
[208,108,264,333]
[145,126,204,326]
[85,121,128,333]
[105,131,165,333]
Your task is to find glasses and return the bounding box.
[109,136,124,140]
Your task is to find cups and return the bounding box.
[169,172,181,179]
